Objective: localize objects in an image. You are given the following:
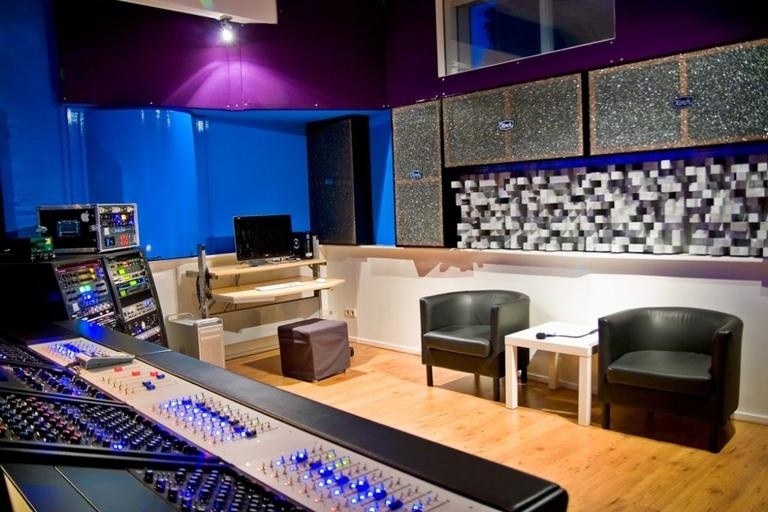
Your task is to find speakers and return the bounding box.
[391,98,456,247]
[304,113,373,246]
[586,37,767,156]
[441,70,587,169]
[293,231,314,260]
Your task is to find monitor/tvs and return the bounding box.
[232,214,294,267]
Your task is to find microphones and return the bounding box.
[535,328,599,339]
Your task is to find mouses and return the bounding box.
[316,278,326,283]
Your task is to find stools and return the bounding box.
[276,316,350,383]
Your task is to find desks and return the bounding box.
[191,235,346,321]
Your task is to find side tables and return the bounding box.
[504,322,599,426]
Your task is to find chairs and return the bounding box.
[597,307,744,454]
[417,290,529,401]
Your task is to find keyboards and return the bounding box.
[255,281,303,291]
